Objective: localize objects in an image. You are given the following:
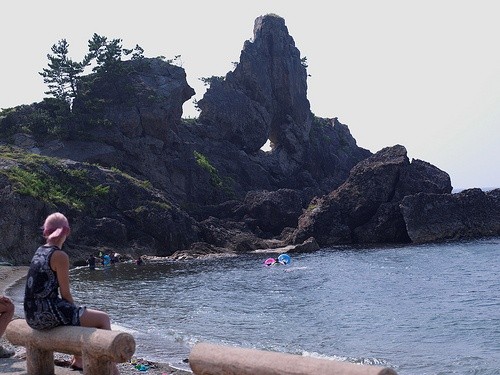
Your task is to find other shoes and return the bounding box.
[70,365,83,371]
[0,343,15,358]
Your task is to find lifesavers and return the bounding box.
[101,255,111,264]
[278,254,290,264]
[264,258,275,266]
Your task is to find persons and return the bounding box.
[267,257,287,267]
[86,250,150,270]
[0,296,15,358]
[23,212,121,375]
